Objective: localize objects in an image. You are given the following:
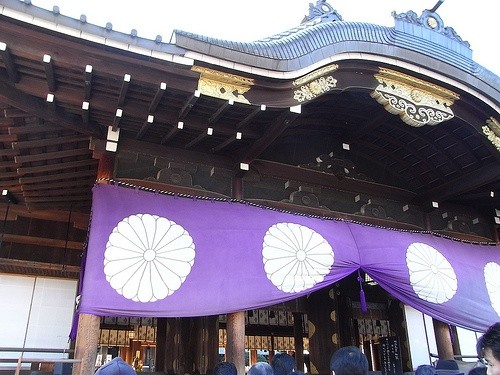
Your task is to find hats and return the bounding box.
[94,357,137,374]
[435,360,465,375]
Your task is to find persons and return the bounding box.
[414,359,487,374]
[475,322,500,375]
[94,356,154,375]
[330,346,369,375]
[210,351,311,375]
[469,334,489,375]
